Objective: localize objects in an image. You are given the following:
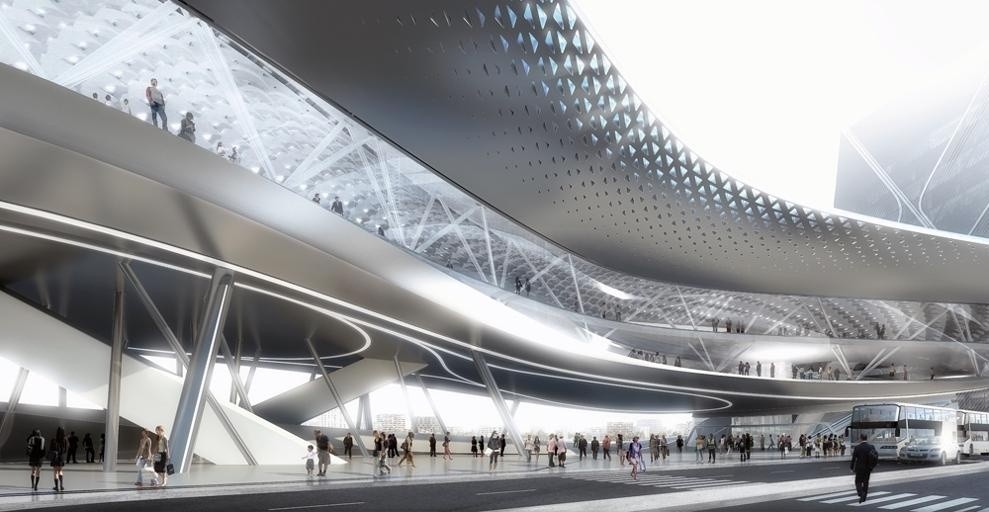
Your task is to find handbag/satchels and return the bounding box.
[166,460,175,476]
[153,453,162,462]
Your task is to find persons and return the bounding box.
[134,426,155,488]
[849,434,879,506]
[446,259,454,269]
[153,426,172,485]
[573,294,626,323]
[510,273,533,299]
[736,361,844,381]
[889,362,938,383]
[628,347,685,366]
[707,319,890,340]
[307,192,388,238]
[93,76,228,155]
[19,425,107,496]
